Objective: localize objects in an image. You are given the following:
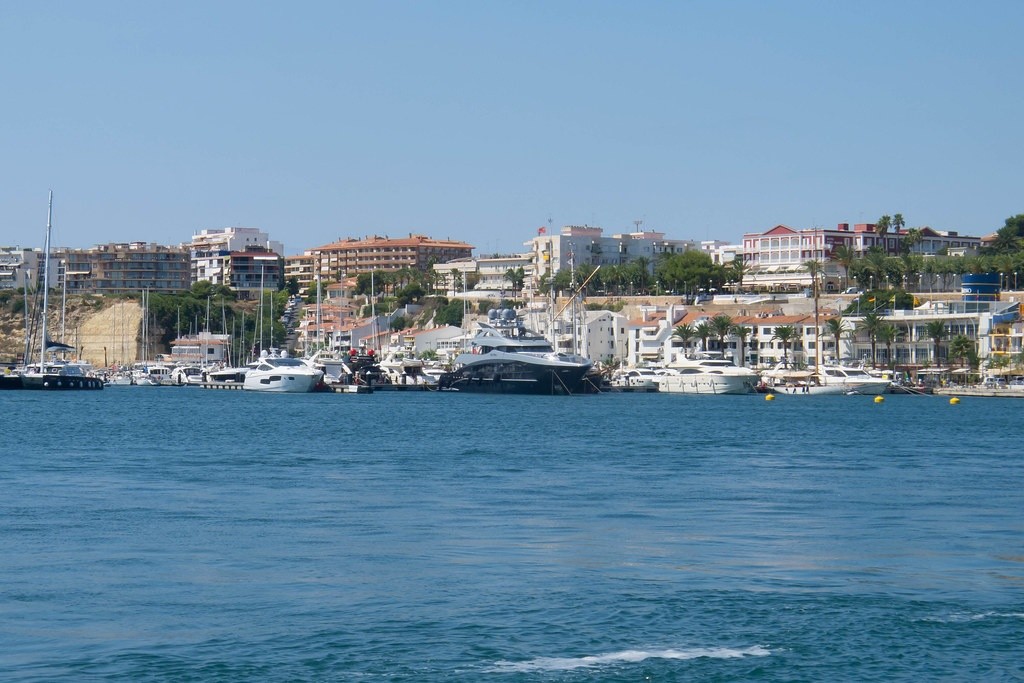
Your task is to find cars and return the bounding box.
[843,287,860,294]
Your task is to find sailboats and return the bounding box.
[0,191,934,397]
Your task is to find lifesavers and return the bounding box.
[51,368,58,373]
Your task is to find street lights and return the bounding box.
[1014,272,1018,292]
[631,281,633,296]
[885,275,889,290]
[919,274,923,293]
[870,275,874,291]
[838,276,841,294]
[953,274,958,293]
[656,281,660,297]
[603,282,606,296]
[937,274,940,292]
[1000,273,1004,292]
